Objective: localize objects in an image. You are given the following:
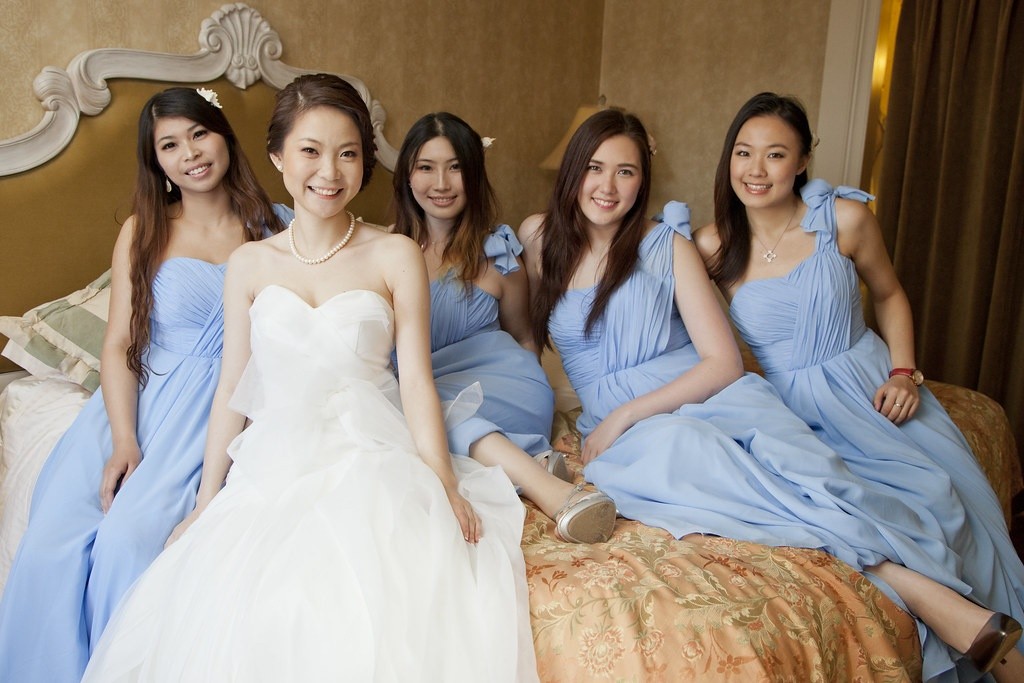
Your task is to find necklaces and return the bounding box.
[289,208,356,265]
[745,197,800,264]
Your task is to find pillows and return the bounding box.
[1,268,115,397]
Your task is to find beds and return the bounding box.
[0,366,1020,683]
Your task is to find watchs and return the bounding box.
[886,366,925,388]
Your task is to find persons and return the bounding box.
[511,96,1024,680]
[76,72,547,681]
[687,89,1023,658]
[378,110,622,545]
[0,85,303,683]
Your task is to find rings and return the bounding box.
[893,401,903,407]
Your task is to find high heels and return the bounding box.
[552,483,623,544]
[533,449,570,482]
[942,597,1023,674]
